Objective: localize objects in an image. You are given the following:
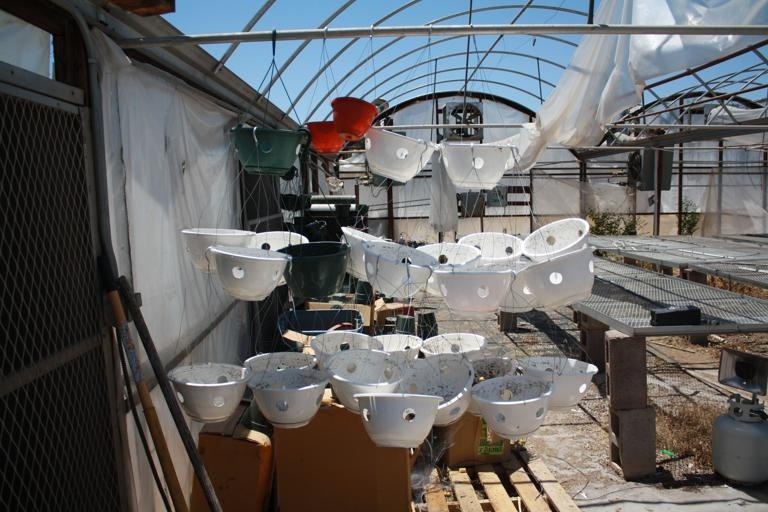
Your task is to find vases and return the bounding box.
[461,357,517,418]
[403,353,472,429]
[358,239,439,298]
[468,373,554,439]
[456,230,521,267]
[410,241,481,295]
[421,330,490,357]
[246,229,309,287]
[205,244,293,301]
[168,359,250,426]
[516,243,597,310]
[339,224,388,287]
[361,124,441,187]
[519,354,597,414]
[272,238,352,300]
[241,349,317,373]
[492,260,536,315]
[325,347,405,415]
[311,329,385,370]
[520,217,592,260]
[330,95,380,140]
[225,123,305,178]
[432,262,513,318]
[249,367,333,430]
[351,392,445,449]
[305,119,346,155]
[178,224,255,279]
[435,138,517,193]
[370,332,421,362]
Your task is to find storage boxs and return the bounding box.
[267,401,416,510]
[192,403,272,507]
[440,413,510,470]
